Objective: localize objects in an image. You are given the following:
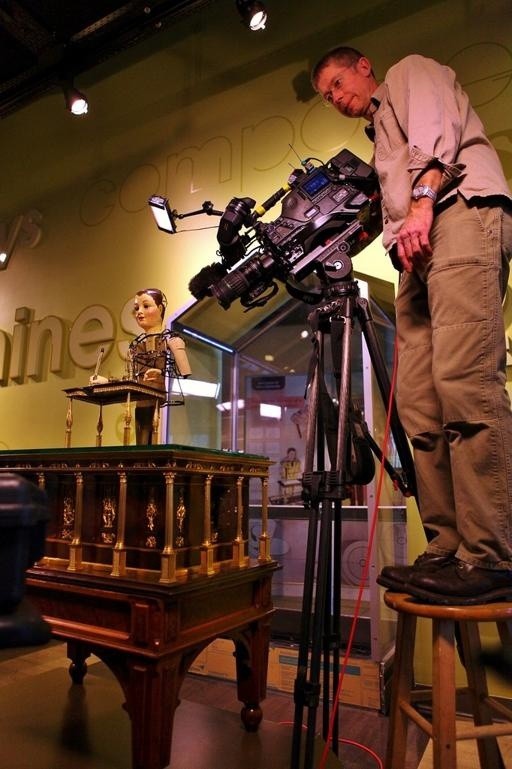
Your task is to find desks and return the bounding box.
[24,563,283,768]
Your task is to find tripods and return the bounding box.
[288,298,507,769]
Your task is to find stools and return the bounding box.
[382,589,512,769]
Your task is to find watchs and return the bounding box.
[410,184,439,201]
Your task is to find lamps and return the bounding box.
[40,0,269,116]
[150,192,222,234]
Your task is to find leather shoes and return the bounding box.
[376,551,512,606]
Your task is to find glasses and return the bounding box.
[322,60,358,107]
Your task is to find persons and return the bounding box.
[88,288,192,383]
[313,49,512,606]
[281,448,302,503]
